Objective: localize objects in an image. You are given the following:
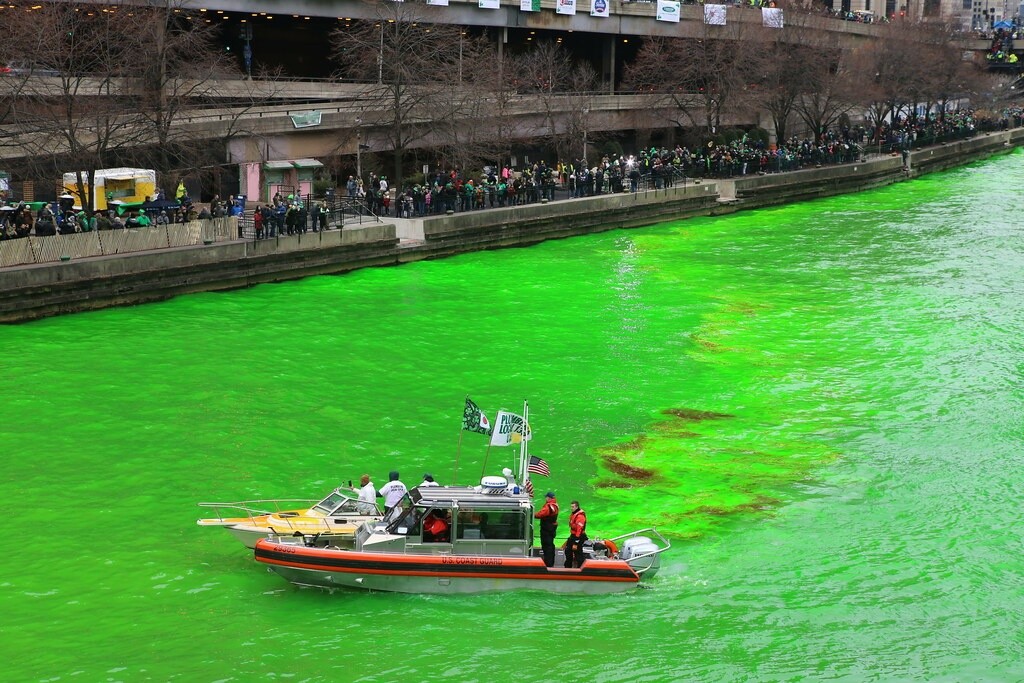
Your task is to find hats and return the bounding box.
[433,509,443,518]
[544,492,555,498]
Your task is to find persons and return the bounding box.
[800,3,929,33]
[948,25,1024,63]
[867,107,978,147]
[1011,9,1021,23]
[423,509,448,535]
[349,471,439,515]
[534,492,559,568]
[564,501,586,568]
[341,144,703,218]
[0,179,333,240]
[680,0,775,9]
[980,105,1024,131]
[705,122,865,178]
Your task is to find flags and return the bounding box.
[463,398,550,477]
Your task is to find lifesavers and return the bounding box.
[596,540,618,561]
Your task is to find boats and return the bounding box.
[195,393,671,596]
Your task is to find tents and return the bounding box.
[62,166,158,211]
[994,20,1012,31]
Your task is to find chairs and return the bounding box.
[430,528,451,542]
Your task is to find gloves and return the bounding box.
[573,538,580,545]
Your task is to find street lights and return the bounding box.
[357,142,370,178]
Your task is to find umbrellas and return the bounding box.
[141,198,179,211]
